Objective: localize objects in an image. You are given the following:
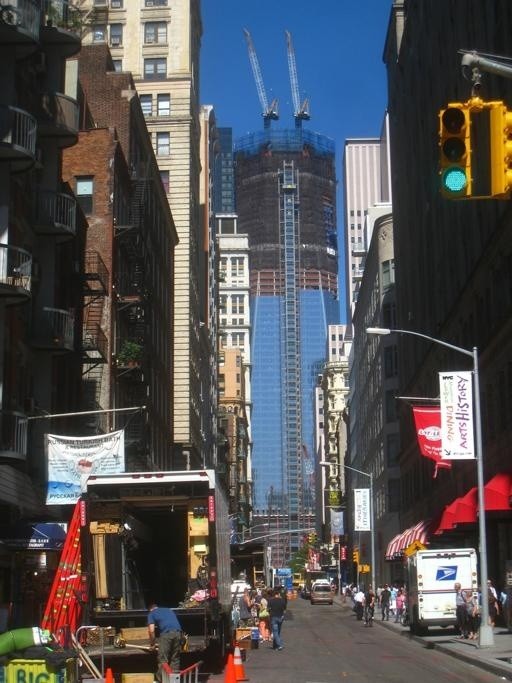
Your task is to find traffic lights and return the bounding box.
[489,102,511,196]
[353,552,357,562]
[440,107,471,196]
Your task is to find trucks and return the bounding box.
[401,550,478,634]
[76,469,230,668]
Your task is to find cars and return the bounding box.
[301,578,334,605]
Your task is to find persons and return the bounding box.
[454,579,508,639]
[146,603,183,683]
[238,583,288,651]
[331,581,406,628]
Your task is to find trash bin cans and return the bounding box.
[3,658,76,683]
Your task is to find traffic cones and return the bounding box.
[224,653,237,683]
[232,640,251,680]
[105,668,114,683]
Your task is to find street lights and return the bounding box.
[239,523,269,535]
[318,459,379,602]
[364,326,497,647]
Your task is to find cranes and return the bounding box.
[281,28,312,127]
[241,26,280,129]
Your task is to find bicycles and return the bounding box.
[363,604,375,627]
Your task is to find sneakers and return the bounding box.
[456,633,480,640]
[273,644,283,651]
